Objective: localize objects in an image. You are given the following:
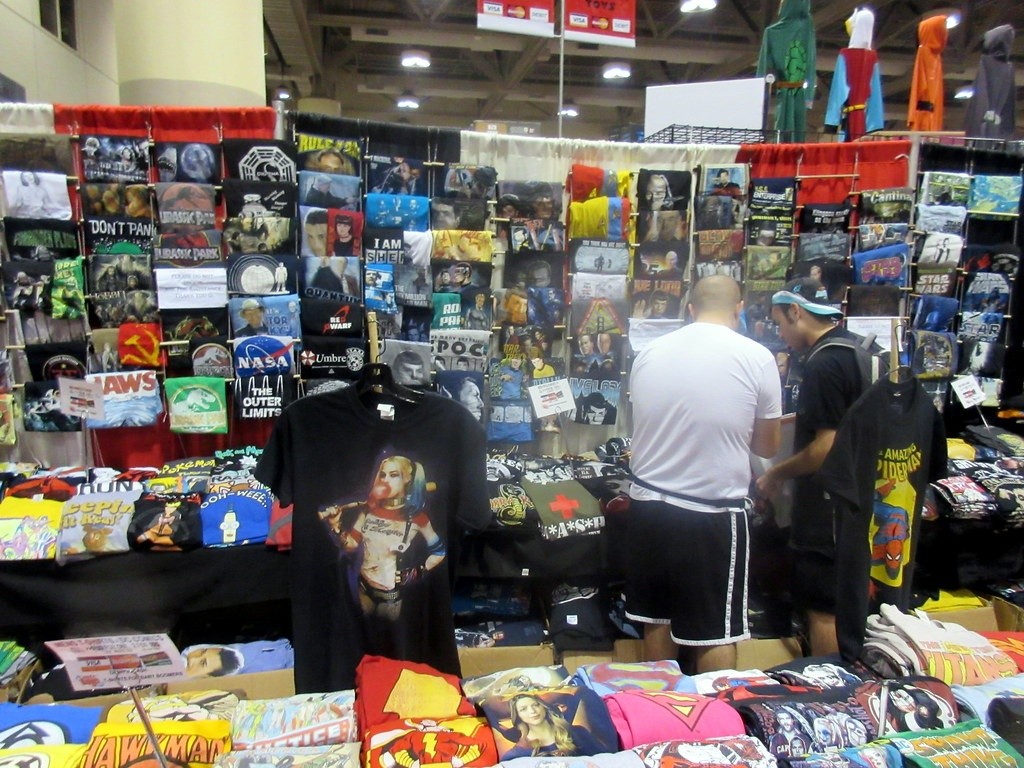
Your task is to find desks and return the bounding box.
[0,520,623,640]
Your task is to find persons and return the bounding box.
[751,277,891,655]
[632,175,682,320]
[583,392,608,424]
[709,170,742,196]
[365,193,489,422]
[934,236,951,266]
[770,663,944,768]
[16,136,360,375]
[576,333,612,355]
[326,455,446,659]
[624,276,782,673]
[752,219,828,299]
[186,647,243,677]
[502,695,606,761]
[501,182,562,325]
[498,326,555,400]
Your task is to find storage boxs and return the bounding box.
[14,658,297,736]
[454,591,1024,689]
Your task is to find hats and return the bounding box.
[772,277,844,319]
[240,299,265,319]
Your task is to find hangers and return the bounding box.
[353,318,424,407]
[882,323,917,379]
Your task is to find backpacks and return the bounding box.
[803,331,891,390]
